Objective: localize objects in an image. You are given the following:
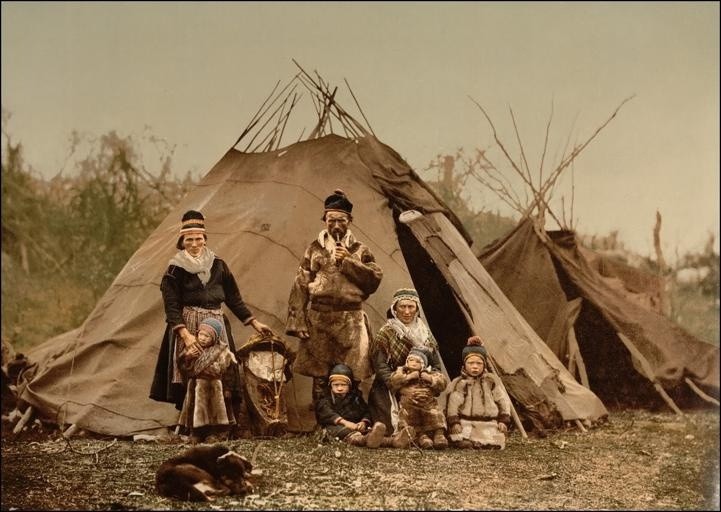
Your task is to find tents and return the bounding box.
[0,132,611,432]
[471,220,720,413]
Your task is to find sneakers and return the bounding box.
[420,434,433,448]
[434,433,449,448]
[455,439,473,450]
[392,426,418,447]
[368,422,386,448]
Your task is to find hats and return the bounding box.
[461,337,488,370]
[327,366,354,394]
[178,212,206,235]
[390,287,422,322]
[325,190,353,214]
[405,346,432,374]
[194,318,223,343]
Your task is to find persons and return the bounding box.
[369,289,442,437]
[391,348,447,449]
[179,320,239,442]
[315,365,388,447]
[150,208,273,441]
[287,189,381,421]
[447,337,510,449]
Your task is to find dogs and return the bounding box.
[155,444,254,503]
